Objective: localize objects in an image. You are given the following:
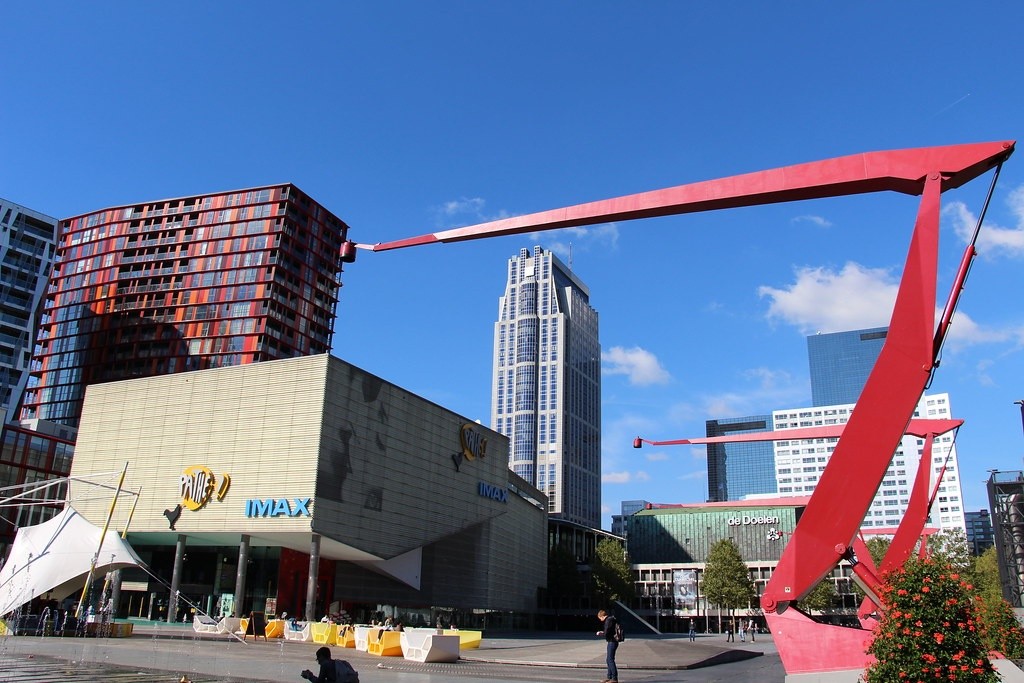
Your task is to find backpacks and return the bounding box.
[329,659,360,683]
[607,617,625,642]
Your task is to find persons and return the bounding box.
[321,615,329,624]
[437,614,444,628]
[738,619,756,643]
[372,613,404,628]
[301,647,360,683]
[449,612,456,629]
[726,620,734,642]
[688,619,696,642]
[596,610,620,683]
[201,612,235,625]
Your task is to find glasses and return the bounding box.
[316,657,319,661]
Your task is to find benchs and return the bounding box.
[193,615,483,663]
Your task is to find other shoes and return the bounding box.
[732,641,734,642]
[606,679,618,683]
[751,640,755,643]
[600,679,610,683]
[742,640,745,642]
[727,641,729,642]
